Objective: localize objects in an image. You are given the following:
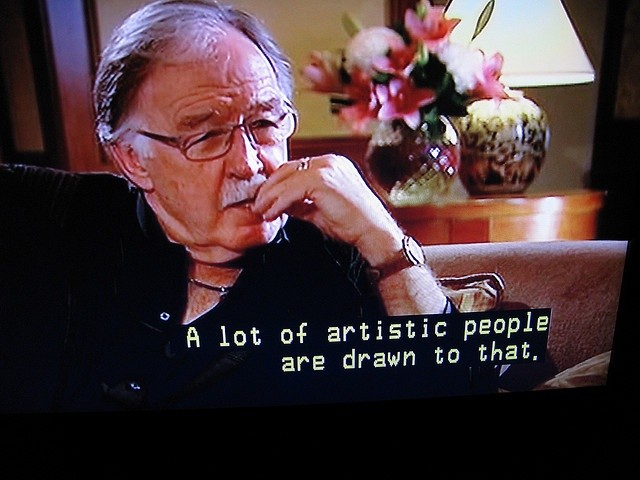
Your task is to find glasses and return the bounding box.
[127,100,297,162]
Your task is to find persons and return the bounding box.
[1,2,482,397]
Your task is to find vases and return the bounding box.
[363,116,463,209]
[453,90,552,199]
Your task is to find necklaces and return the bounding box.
[187,277,233,291]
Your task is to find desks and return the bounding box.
[296,138,614,246]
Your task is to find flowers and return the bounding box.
[298,1,525,138]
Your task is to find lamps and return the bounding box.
[444,0,597,88]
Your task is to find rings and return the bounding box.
[297,156,310,171]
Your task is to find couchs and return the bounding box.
[411,240,630,394]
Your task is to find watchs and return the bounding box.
[365,235,431,285]
